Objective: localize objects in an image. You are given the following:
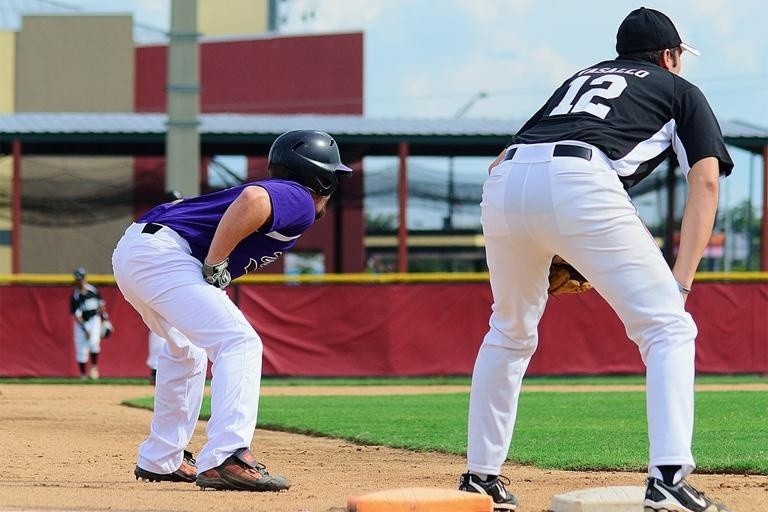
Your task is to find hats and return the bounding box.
[73,268,86,280]
[616,7,700,57]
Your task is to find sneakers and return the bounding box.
[135,449,197,483]
[195,447,292,491]
[643,476,730,512]
[82,368,99,379]
[459,473,518,512]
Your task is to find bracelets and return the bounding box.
[676,281,691,293]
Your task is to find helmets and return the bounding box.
[267,129,353,198]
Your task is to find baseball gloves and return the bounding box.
[548,255,594,295]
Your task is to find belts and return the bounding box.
[502,144,592,161]
[142,223,162,234]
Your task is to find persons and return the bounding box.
[112,130,354,492]
[146,332,165,385]
[458,6,734,512]
[70,267,114,381]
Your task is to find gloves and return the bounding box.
[202,258,231,289]
